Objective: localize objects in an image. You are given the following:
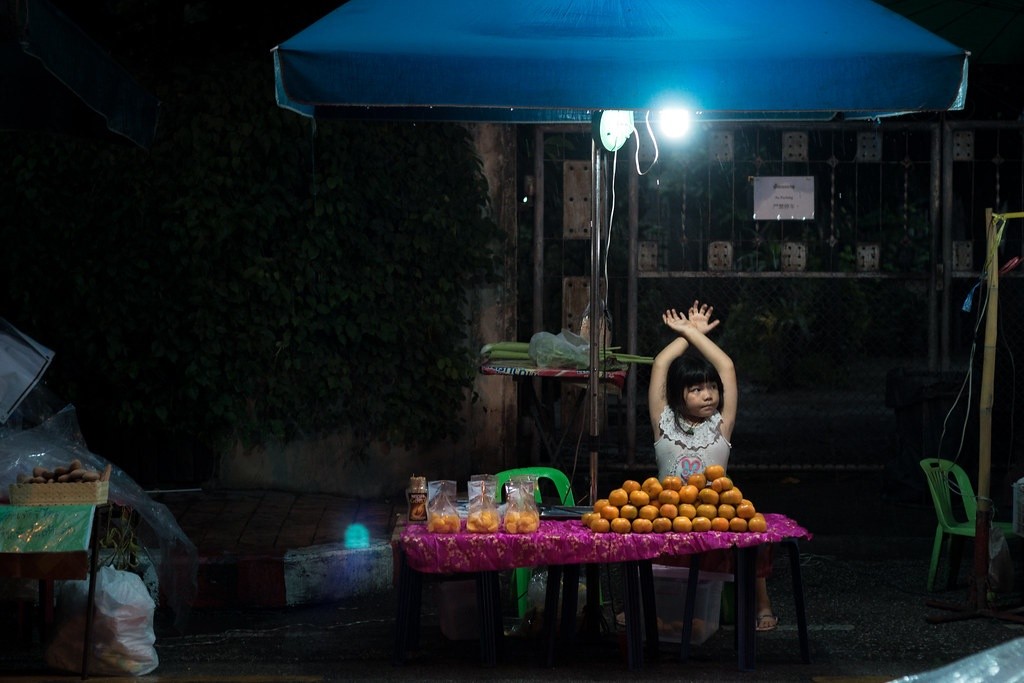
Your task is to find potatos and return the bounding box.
[17,460,101,483]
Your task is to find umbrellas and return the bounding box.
[268,0,969,641]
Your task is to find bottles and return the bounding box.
[405,476,429,525]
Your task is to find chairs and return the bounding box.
[495,468,603,620]
[920,458,1018,603]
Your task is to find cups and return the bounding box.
[502,616,523,638]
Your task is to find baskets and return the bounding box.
[9,464,111,504]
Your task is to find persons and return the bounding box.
[613,299,777,631]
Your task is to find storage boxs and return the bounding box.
[1013,477,1024,538]
[637,563,733,647]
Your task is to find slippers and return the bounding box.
[754,613,777,630]
[615,611,625,626]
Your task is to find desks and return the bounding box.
[481,366,626,487]
[0,502,139,681]
[391,514,814,672]
